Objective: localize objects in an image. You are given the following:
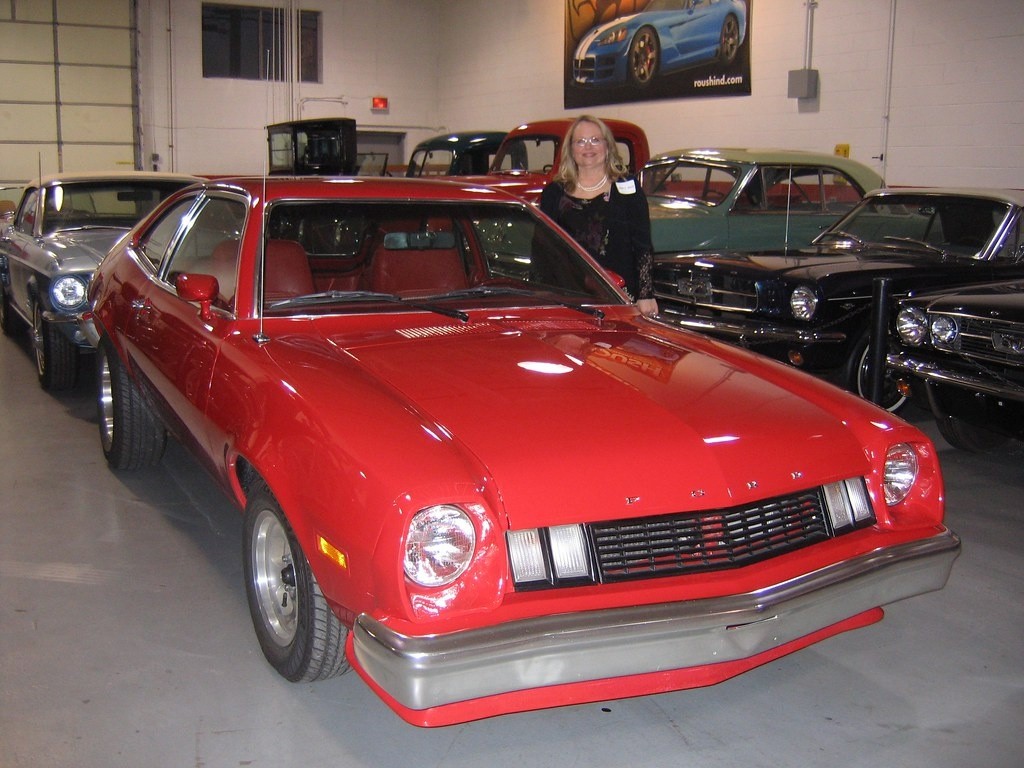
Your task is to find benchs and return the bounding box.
[192,254,364,292]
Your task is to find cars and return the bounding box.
[79,173,963,731]
[647,186,1024,417]
[1,171,241,395]
[880,276,1024,456]
[263,119,949,285]
[567,0,747,93]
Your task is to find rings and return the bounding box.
[650,312,655,315]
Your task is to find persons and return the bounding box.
[529,116,659,317]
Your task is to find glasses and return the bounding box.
[570,136,605,146]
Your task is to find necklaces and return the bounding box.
[577,174,607,191]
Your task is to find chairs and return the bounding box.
[210,240,314,309]
[373,222,468,299]
